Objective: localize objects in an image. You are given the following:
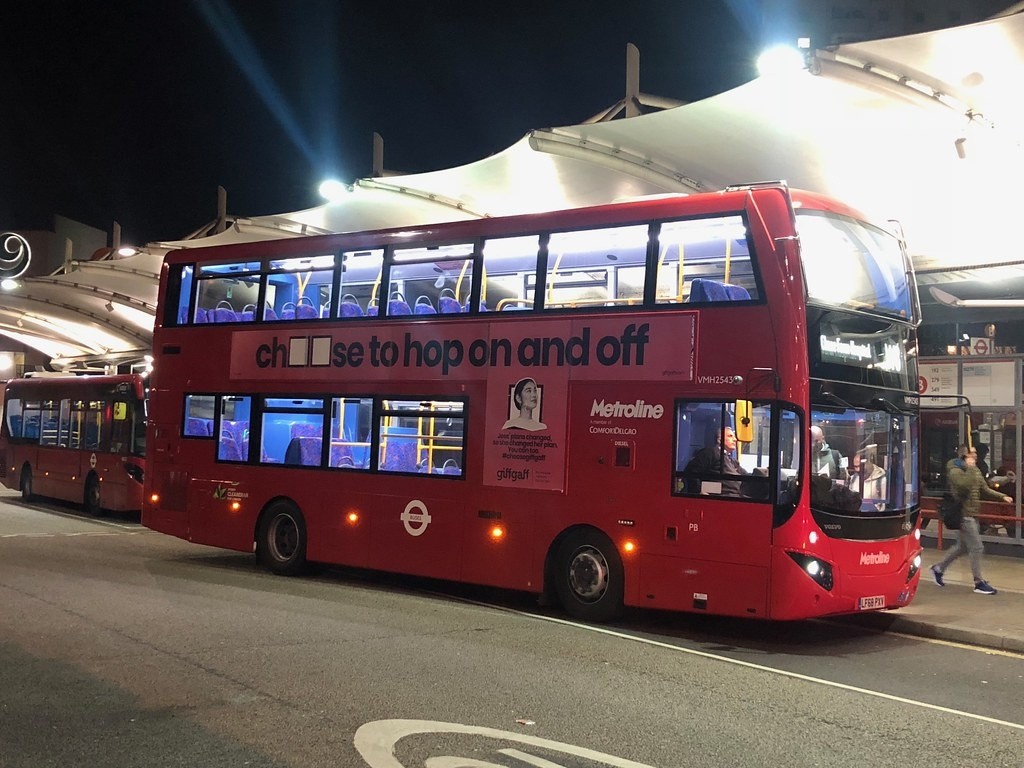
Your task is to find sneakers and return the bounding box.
[973,580,997,595]
[928,564,945,587]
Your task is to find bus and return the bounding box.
[0,374,149,518]
[142,179,979,624]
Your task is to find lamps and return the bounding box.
[749,40,826,87]
[1,279,23,289]
[115,242,141,259]
[318,175,359,201]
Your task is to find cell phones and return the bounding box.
[961,454,967,460]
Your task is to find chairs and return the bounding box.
[323,301,332,317]
[365,298,380,317]
[281,302,296,320]
[363,458,380,469]
[214,301,238,322]
[240,303,256,321]
[464,294,488,312]
[413,295,437,314]
[252,300,278,320]
[10,414,99,448]
[235,422,279,462]
[340,293,364,316]
[441,459,461,475]
[335,456,354,468]
[207,420,233,437]
[418,458,437,474]
[221,430,242,460]
[438,288,462,312]
[295,297,319,319]
[389,291,413,315]
[242,429,267,461]
[502,304,514,310]
[188,417,208,435]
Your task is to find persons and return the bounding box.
[929,443,1013,594]
[931,345,946,356]
[921,444,1016,497]
[844,447,886,512]
[684,425,751,498]
[502,377,547,431]
[797,426,848,480]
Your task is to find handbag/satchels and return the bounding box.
[939,491,962,530]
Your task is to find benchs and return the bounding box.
[283,436,353,469]
[689,278,753,300]
[182,306,242,323]
[363,426,438,475]
[289,421,354,456]
